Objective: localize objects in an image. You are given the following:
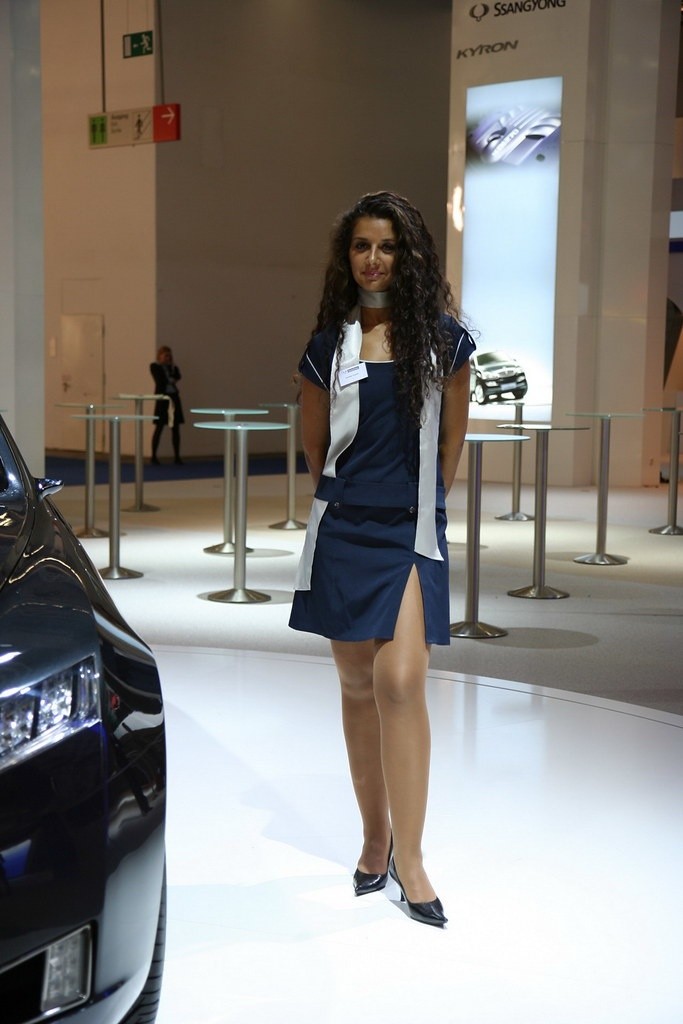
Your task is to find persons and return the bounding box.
[288,190,475,924]
[149,346,185,467]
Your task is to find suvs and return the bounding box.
[470,348,528,406]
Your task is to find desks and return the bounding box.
[53,389,683,639]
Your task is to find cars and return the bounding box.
[0,414,170,1024]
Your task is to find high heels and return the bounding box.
[353,828,394,894]
[389,855,448,924]
